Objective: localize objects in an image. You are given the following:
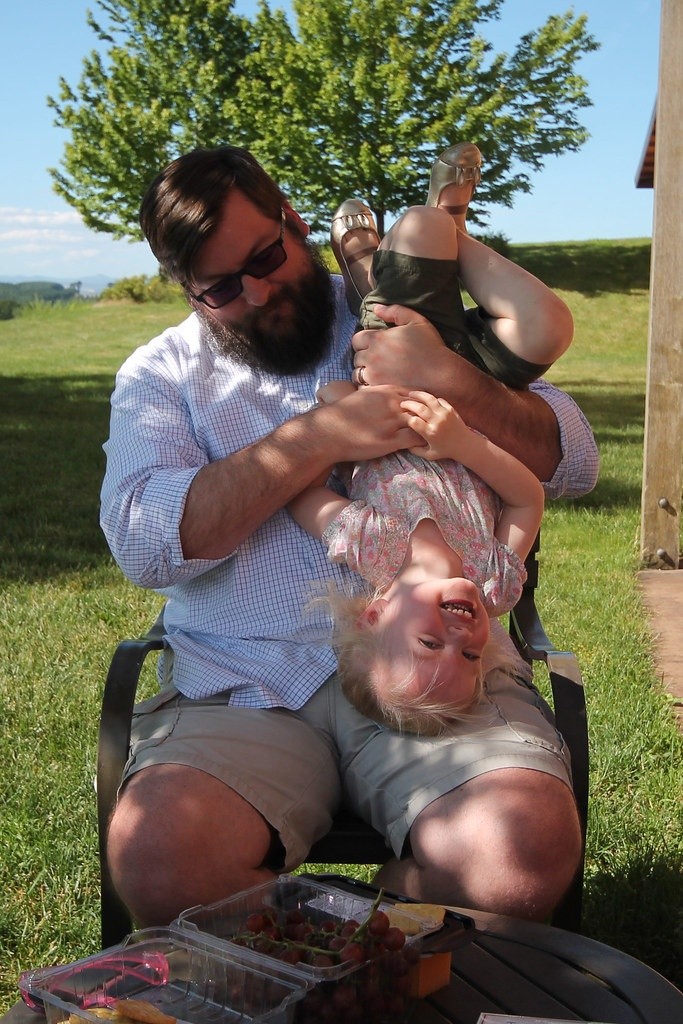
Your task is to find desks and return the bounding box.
[0,907,683,1024]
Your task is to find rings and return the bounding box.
[358,367,369,386]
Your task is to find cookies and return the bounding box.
[382,911,419,934]
[394,902,446,922]
[69,999,177,1024]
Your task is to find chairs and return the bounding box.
[99,531,588,950]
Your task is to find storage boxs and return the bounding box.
[268,872,475,996]
[17,873,445,1024]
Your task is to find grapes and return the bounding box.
[204,908,422,1024]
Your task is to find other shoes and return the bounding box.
[424,141,480,231]
[330,198,382,316]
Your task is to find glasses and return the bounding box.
[180,204,288,310]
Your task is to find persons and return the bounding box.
[99,147,599,933]
[284,143,574,734]
